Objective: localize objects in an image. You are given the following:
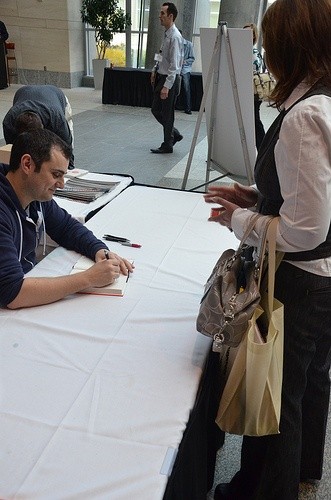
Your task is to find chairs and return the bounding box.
[5,42,19,87]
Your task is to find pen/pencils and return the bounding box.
[122,243,142,247]
[104,250,109,259]
[103,234,130,242]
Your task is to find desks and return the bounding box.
[0,183,248,500]
[101,67,203,112]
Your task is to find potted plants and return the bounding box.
[80,0,132,91]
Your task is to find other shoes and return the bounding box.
[185,109,192,114]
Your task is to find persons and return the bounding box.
[151,1,184,154]
[2,83,74,167]
[203,1,330,500]
[241,22,266,158]
[0,21,9,91]
[173,28,196,114]
[0,128,136,310]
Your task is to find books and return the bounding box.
[66,254,136,297]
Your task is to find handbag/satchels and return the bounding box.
[254,73,281,101]
[214,215,285,437]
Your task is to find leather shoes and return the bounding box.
[172,136,183,146]
[150,148,174,154]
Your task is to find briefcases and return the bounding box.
[197,213,271,353]
